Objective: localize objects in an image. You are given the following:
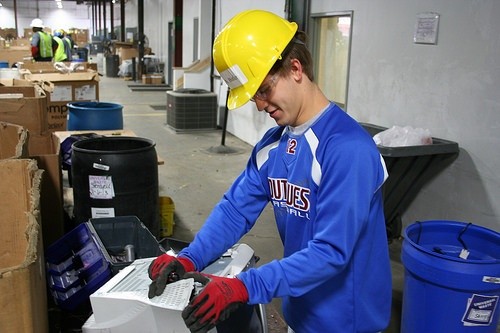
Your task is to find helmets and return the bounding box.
[66,31,72,37]
[54,29,65,38]
[30,18,45,28]
[213,8,298,110]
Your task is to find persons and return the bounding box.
[51,30,77,61]
[30,18,59,62]
[149,9,394,333]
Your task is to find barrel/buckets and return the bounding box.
[401,220,500,333]
[67,102,123,130]
[71,136,160,240]
[106,55,119,77]
[158,197,175,237]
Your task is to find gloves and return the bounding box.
[148,253,194,298]
[182,271,248,333]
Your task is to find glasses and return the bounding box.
[250,59,288,102]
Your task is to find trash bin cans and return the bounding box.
[106,54,120,78]
